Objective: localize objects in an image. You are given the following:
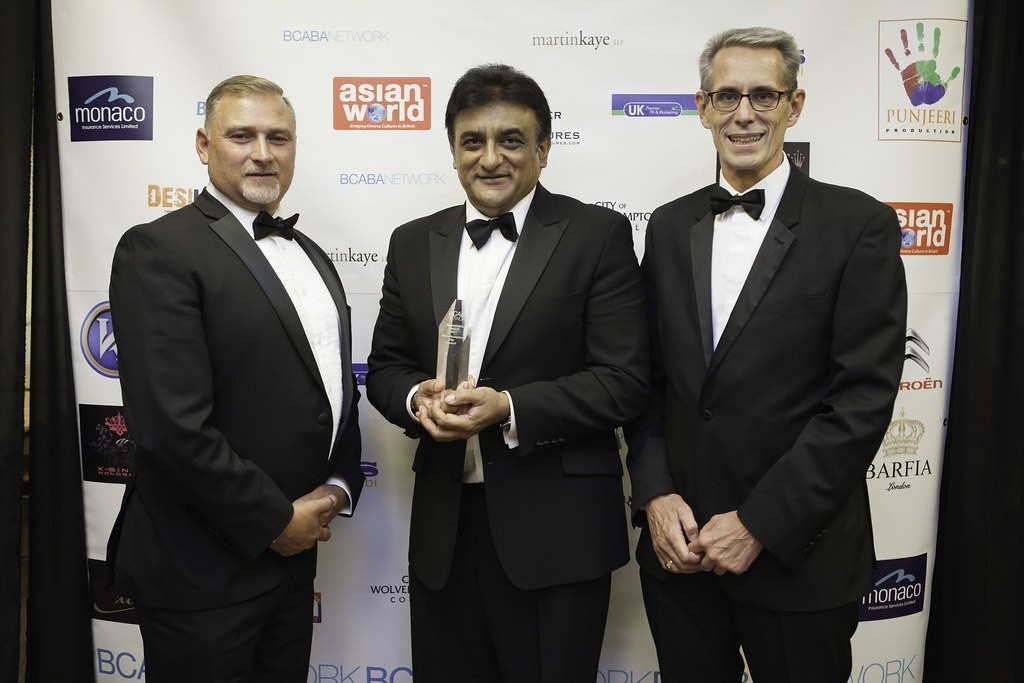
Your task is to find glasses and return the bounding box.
[703,90,791,113]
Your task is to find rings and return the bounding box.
[665,559,674,570]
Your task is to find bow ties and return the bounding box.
[710,184,765,221]
[253,211,299,242]
[464,212,519,250]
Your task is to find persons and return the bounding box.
[105,76,364,683]
[625,31,908,683]
[366,64,650,683]
